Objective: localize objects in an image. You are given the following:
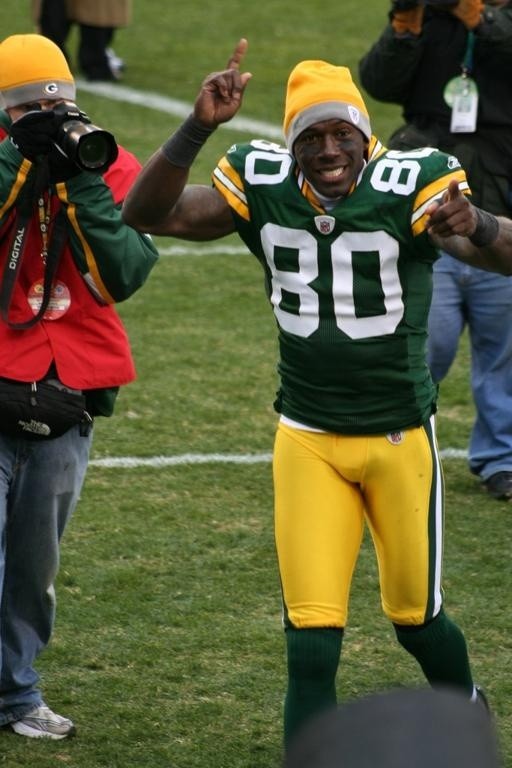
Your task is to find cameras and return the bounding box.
[50,100,119,177]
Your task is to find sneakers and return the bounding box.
[485,470,512,501]
[54,43,124,81]
[476,686,490,715]
[9,702,76,741]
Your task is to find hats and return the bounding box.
[0,33,76,110]
[283,59,372,161]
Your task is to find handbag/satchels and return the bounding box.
[0,378,85,441]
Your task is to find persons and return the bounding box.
[32,0,132,81]
[123,33,511,768]
[355,1,511,501]
[0,42,159,741]
[292,684,508,767]
